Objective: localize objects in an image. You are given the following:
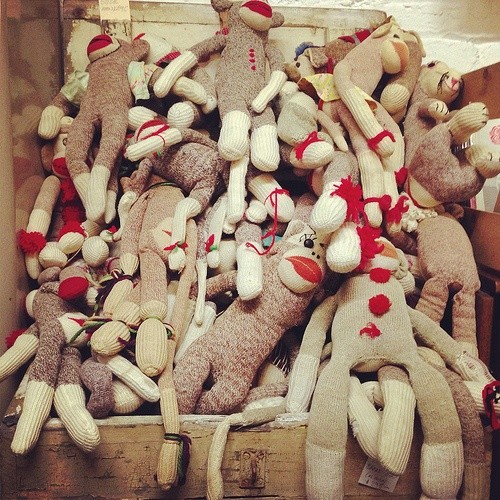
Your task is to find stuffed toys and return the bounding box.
[0,0,500,500]
